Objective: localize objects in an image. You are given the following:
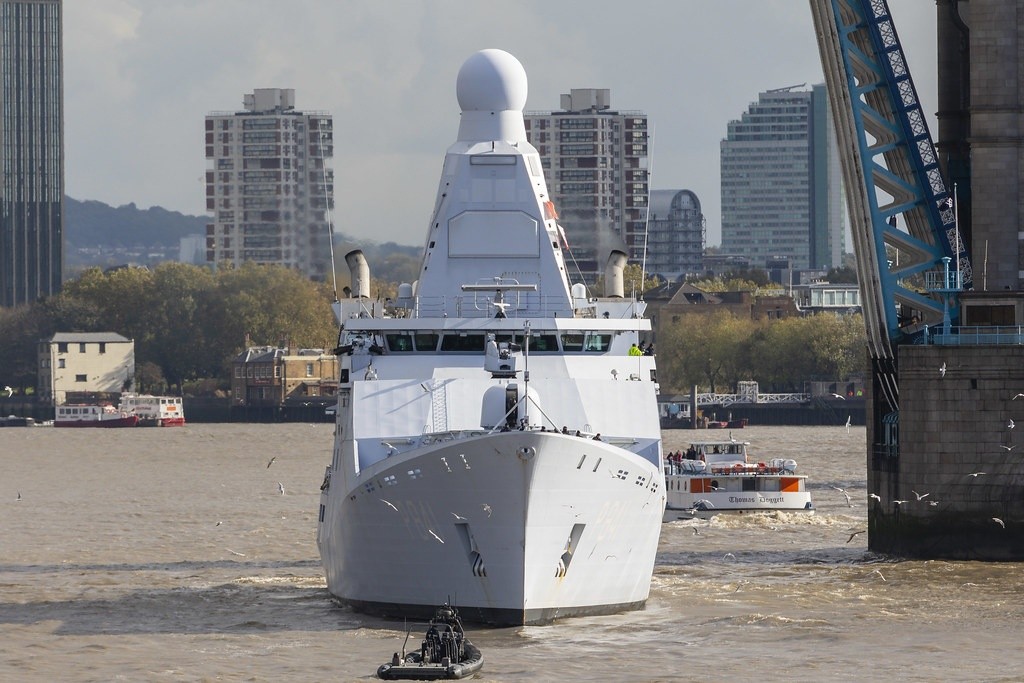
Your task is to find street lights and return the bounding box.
[941,256,953,290]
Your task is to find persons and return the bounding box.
[666,446,696,465]
[426,624,461,658]
[628,343,642,356]
[638,340,649,356]
[857,388,863,396]
[498,418,602,441]
[848,389,854,397]
[646,343,657,356]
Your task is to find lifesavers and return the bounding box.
[111,408,116,413]
[106,409,111,414]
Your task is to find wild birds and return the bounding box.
[226,548,245,558]
[877,570,886,582]
[692,526,700,535]
[967,472,987,477]
[1000,445,1016,451]
[605,555,616,560]
[383,441,400,454]
[641,495,651,509]
[609,468,619,478]
[303,402,311,406]
[451,513,467,521]
[1007,419,1016,429]
[428,529,444,543]
[278,481,285,496]
[707,486,726,490]
[940,363,947,378]
[832,393,852,428]
[483,503,493,518]
[686,499,715,514]
[267,457,276,467]
[893,500,909,504]
[913,491,929,501]
[1012,393,1024,400]
[371,495,398,511]
[924,500,939,506]
[847,531,866,543]
[364,359,374,380]
[827,485,864,507]
[993,518,1005,528]
[869,493,880,502]
[722,552,735,560]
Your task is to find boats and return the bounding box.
[53,399,141,427]
[118,390,187,427]
[662,429,817,524]
[376,594,484,681]
[312,45,670,628]
